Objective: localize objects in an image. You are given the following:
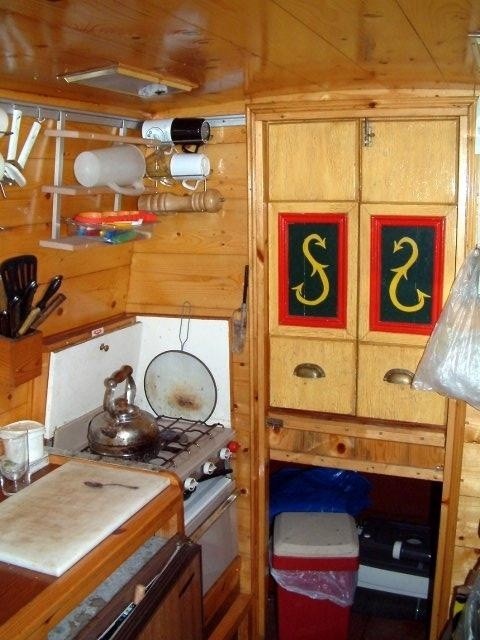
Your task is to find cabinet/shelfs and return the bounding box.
[0,113,141,354]
[429,129,480,640]
[121,124,250,318]
[0,454,205,640]
[246,97,469,640]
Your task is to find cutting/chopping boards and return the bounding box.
[0,460,172,578]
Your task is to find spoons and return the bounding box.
[82,480,144,492]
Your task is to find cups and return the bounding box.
[72,145,148,198]
[141,119,171,143]
[145,146,175,187]
[170,152,209,191]
[171,117,212,153]
[0,426,32,495]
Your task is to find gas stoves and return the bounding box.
[52,393,238,502]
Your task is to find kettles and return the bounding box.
[86,364,162,464]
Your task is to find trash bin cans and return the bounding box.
[272,512,360,640]
